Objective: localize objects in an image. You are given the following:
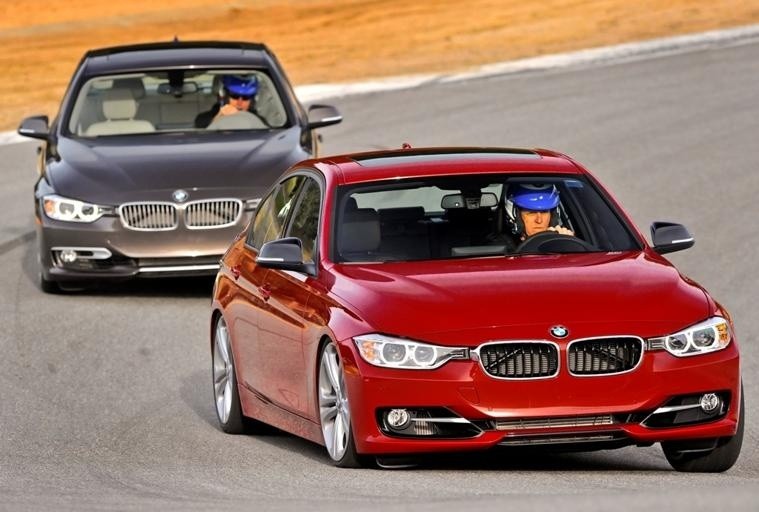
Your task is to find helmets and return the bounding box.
[502,181,562,238]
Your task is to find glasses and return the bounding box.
[228,94,254,100]
[217,74,260,113]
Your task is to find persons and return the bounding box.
[195,85,273,129]
[514,200,575,246]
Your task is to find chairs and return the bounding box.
[69,80,276,147]
[331,202,571,265]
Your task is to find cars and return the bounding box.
[16,34,342,296]
[210,142,745,474]
